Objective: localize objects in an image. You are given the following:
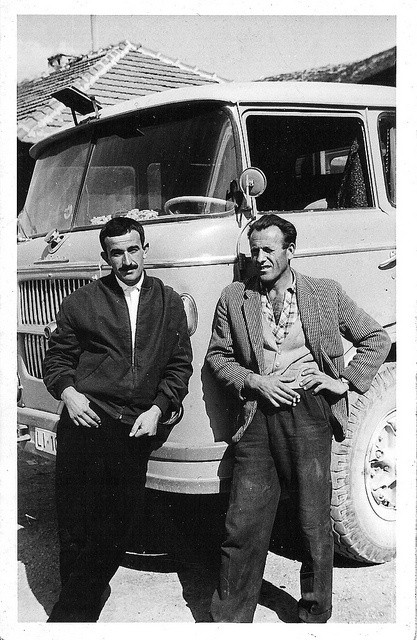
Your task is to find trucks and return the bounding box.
[16,81,398,566]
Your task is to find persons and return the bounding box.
[42,217,194,623]
[204,213,392,624]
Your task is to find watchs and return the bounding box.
[339,377,349,384]
